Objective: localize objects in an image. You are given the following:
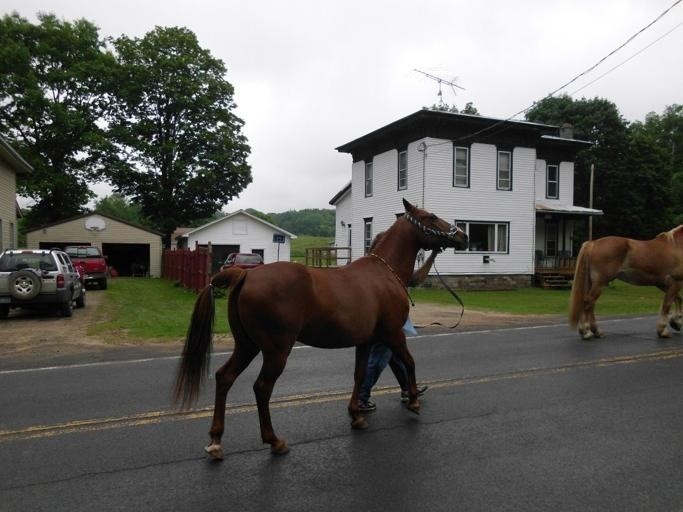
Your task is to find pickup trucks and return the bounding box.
[61,245,108,289]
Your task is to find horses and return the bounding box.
[568,224,683,341]
[169,196,469,461]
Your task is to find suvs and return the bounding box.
[0,247,86,318]
[220,253,264,273]
